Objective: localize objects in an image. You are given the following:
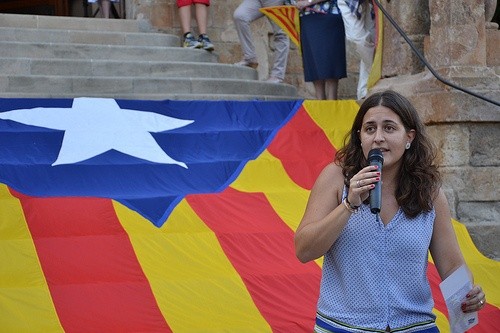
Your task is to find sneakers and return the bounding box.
[182,33,204,48]
[198,34,215,52]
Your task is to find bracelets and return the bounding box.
[344,198,359,213]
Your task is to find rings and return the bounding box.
[480,301,483,304]
[357,179,360,187]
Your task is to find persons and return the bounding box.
[296,89,486,333]
[175,0,215,51]
[86,0,119,19]
[233,0,289,82]
[295,0,376,99]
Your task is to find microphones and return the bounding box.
[368,148,384,215]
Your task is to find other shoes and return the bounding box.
[235,61,259,70]
